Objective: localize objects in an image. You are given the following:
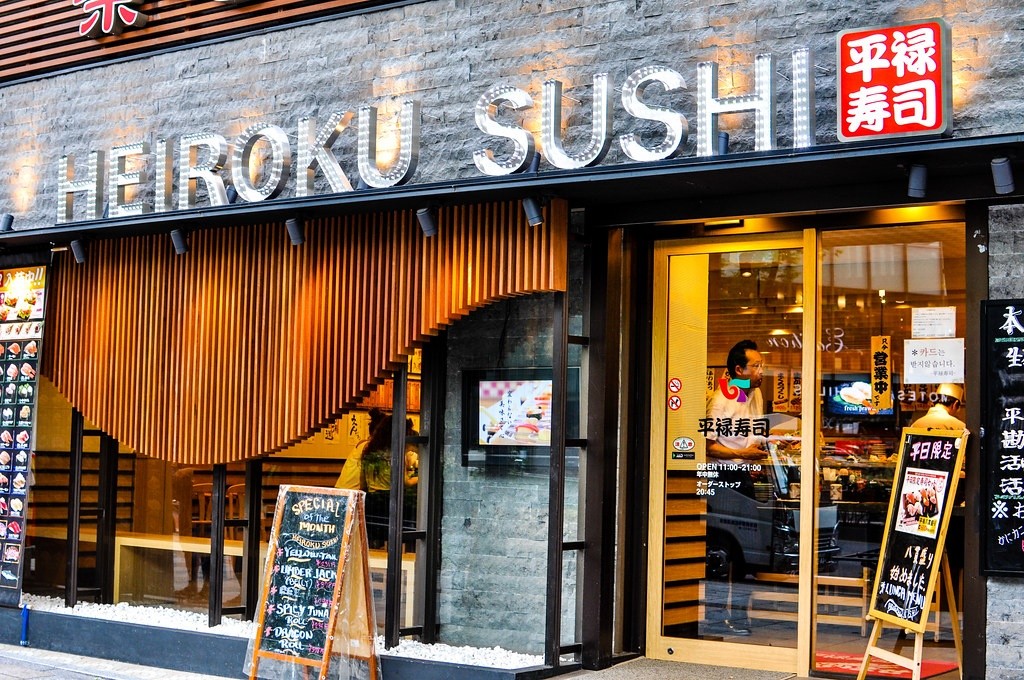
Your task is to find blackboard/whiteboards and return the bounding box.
[256,483,361,669]
[865,425,969,640]
[980,297,1024,580]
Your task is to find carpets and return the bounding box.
[812,651,958,680]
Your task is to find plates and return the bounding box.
[871,443,885,458]
[754,483,773,501]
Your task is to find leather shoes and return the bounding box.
[704,620,751,635]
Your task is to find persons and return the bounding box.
[702,340,797,637]
[910,384,968,630]
[332,409,421,555]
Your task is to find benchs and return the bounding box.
[24,523,415,638]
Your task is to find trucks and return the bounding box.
[703,415,841,580]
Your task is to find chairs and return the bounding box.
[191,481,247,576]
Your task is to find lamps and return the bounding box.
[170,229,189,255]
[525,152,541,174]
[416,207,436,237]
[284,218,306,245]
[226,185,237,204]
[522,198,544,227]
[0,213,14,233]
[908,163,927,198]
[991,157,1015,195]
[70,240,85,264]
[718,132,729,154]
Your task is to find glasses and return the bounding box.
[742,360,765,369]
